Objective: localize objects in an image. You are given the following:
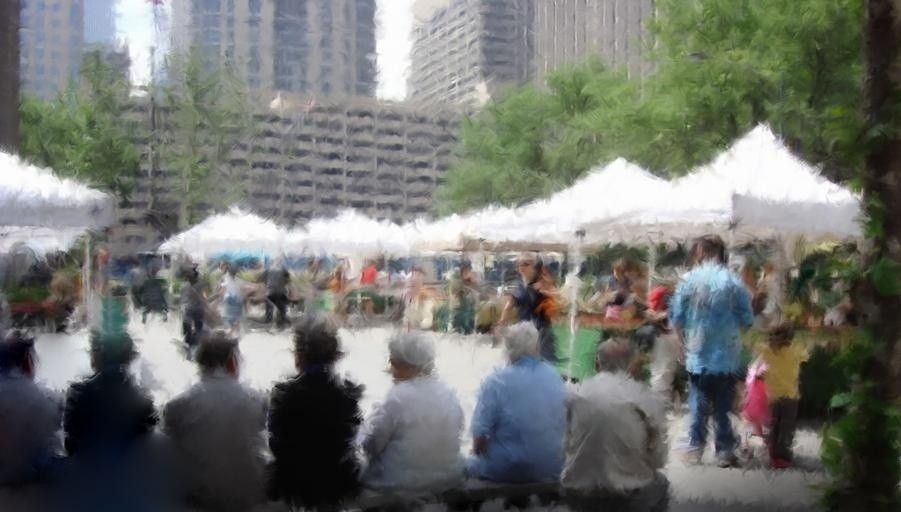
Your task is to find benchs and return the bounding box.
[442,471,559,511]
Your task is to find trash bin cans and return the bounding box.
[551,321,604,384]
[101,294,129,334]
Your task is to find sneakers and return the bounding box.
[683,445,798,469]
